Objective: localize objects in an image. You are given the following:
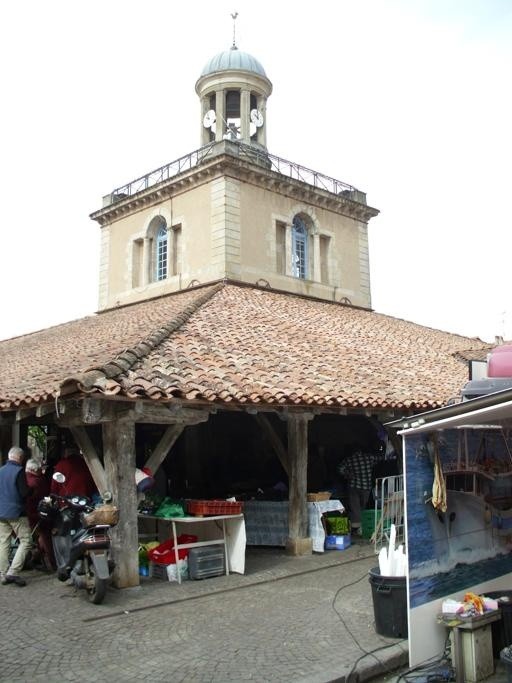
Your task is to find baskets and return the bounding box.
[307,491,332,502]
[184,499,245,515]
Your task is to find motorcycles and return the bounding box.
[47,467,124,606]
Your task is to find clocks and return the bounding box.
[250,105,263,128]
[203,109,216,129]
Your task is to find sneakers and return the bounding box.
[6,574,26,587]
[1,577,12,585]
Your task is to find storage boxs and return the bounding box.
[150,561,171,582]
[184,498,244,518]
[325,534,351,550]
[327,517,349,535]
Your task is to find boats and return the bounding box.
[415,429,512,571]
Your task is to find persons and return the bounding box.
[49,438,100,583]
[334,428,387,536]
[0,445,41,588]
[22,458,58,572]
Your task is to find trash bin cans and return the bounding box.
[478,590,512,658]
[368,566,408,638]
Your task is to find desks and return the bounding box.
[242,500,345,553]
[136,512,247,585]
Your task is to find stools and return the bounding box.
[436,607,502,683]
